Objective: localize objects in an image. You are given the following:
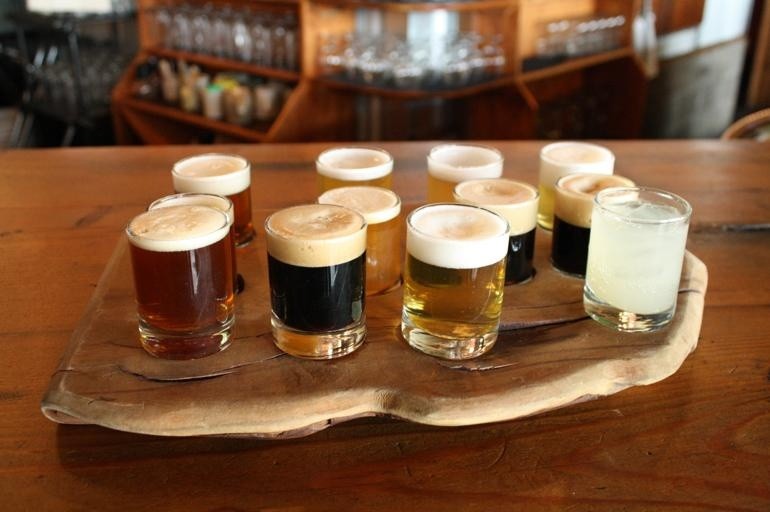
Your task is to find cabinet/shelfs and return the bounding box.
[112,2,353,147]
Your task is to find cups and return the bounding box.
[425,143,506,206]
[579,186,691,332]
[171,153,255,247]
[452,180,540,286]
[317,187,403,295]
[402,202,509,363]
[535,141,616,229]
[315,145,394,193]
[262,204,371,360]
[150,138,637,296]
[549,176,636,281]
[133,58,279,125]
[123,205,234,360]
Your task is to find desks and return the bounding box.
[0,137,768,511]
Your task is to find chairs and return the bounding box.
[0,1,114,148]
